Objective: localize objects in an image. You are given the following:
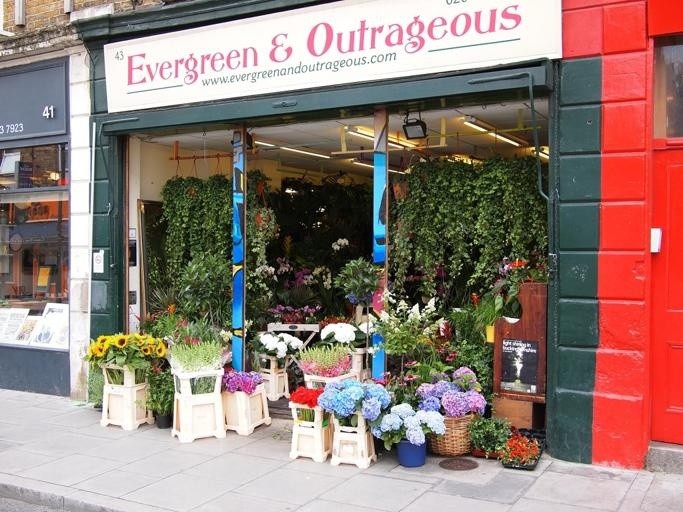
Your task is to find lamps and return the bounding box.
[531,146,549,159]
[350,158,405,174]
[458,114,529,146]
[402,112,427,139]
[253,134,332,159]
[343,126,420,148]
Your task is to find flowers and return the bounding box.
[414,367,490,418]
[289,386,321,407]
[504,433,545,466]
[360,281,446,352]
[85,330,166,366]
[319,380,395,421]
[223,368,262,395]
[382,402,448,446]
[259,332,302,359]
[319,322,358,346]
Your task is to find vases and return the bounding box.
[98,360,154,430]
[255,353,291,401]
[395,439,425,468]
[287,400,333,463]
[221,382,273,434]
[331,411,377,469]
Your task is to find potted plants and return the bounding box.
[143,367,173,429]
[467,416,509,459]
[167,341,230,444]
